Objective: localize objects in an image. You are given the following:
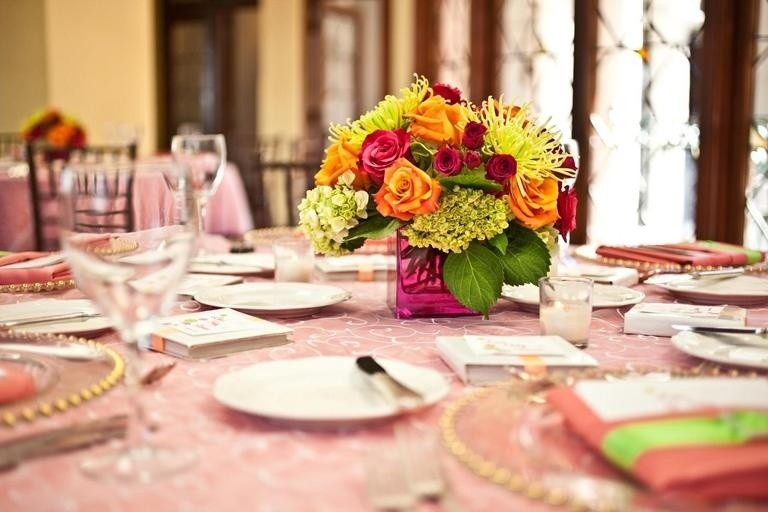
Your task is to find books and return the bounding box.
[427,330,603,387]
[133,304,293,363]
[186,250,277,280]
[620,299,749,341]
[307,253,397,284]
[563,263,641,289]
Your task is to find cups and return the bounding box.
[272,238,316,282]
[536,274,594,351]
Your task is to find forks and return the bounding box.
[669,322,768,340]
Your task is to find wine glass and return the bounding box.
[171,133,228,245]
[53,157,202,487]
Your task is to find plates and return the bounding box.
[185,251,275,275]
[193,280,354,320]
[0,295,115,340]
[668,323,768,371]
[644,269,768,305]
[208,352,454,431]
[499,278,644,312]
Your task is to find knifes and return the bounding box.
[0,310,102,332]
[354,352,426,415]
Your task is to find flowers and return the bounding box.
[22,107,85,160]
[298,71,577,320]
